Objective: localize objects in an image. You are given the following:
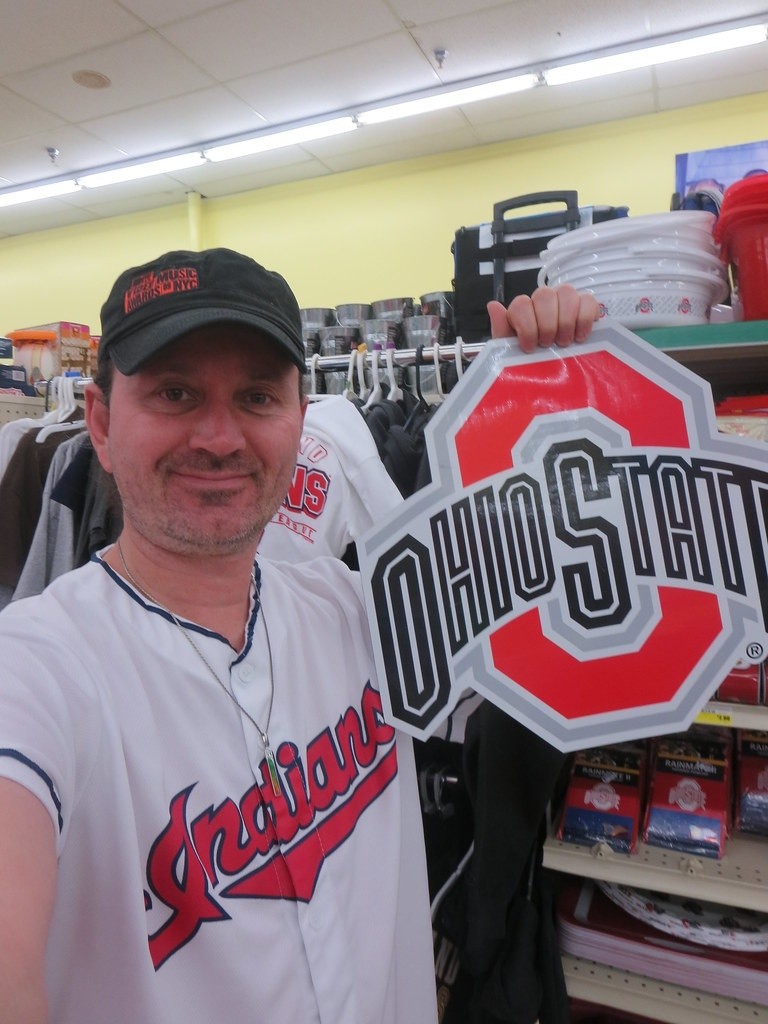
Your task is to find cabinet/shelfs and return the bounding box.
[541,323,768,1024]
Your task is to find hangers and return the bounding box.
[33,376,93,444]
[308,337,466,429]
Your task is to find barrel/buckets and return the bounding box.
[713,174,768,320]
[298,292,471,395]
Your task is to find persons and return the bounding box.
[0,244,602,1024]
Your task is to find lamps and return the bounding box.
[0,13,768,208]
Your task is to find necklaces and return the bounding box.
[113,536,281,795]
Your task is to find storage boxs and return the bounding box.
[444,187,631,343]
[11,322,101,383]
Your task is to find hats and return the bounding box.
[97,248,308,377]
